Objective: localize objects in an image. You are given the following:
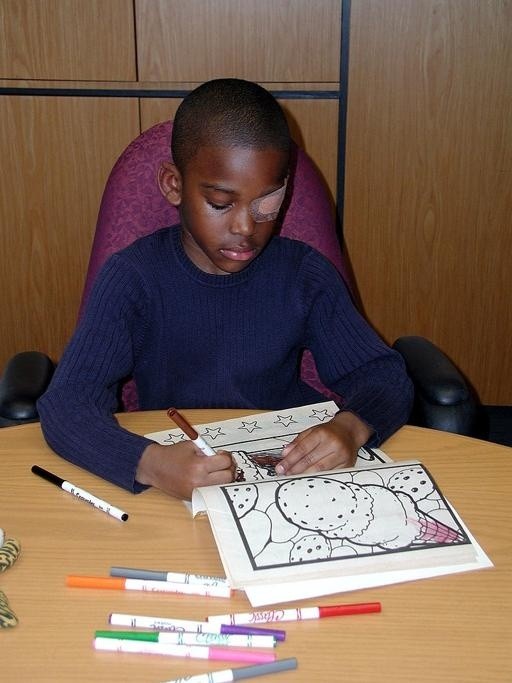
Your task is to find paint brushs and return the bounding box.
[209,602,383,626]
[68,566,231,599]
[163,657,298,683]
[94,614,288,663]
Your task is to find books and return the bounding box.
[143,400,494,608]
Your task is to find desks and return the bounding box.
[1,409,512,678]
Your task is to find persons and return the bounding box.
[36,78,414,502]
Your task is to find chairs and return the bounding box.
[0,121,485,436]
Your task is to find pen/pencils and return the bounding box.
[168,409,240,480]
[31,464,128,522]
